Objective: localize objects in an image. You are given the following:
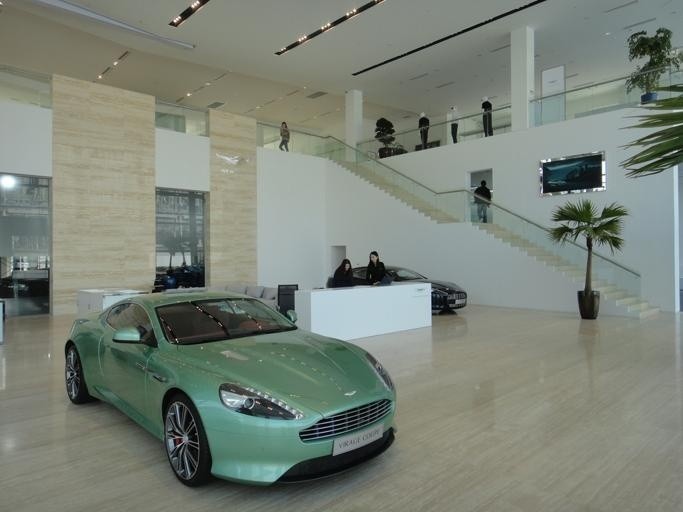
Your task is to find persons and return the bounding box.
[474,180,491,222]
[279,122,290,152]
[334,258,353,282]
[450,106,458,143]
[418,111,430,149]
[365,250,386,285]
[162,270,179,288]
[481,96,493,136]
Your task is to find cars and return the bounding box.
[154,265,205,289]
[326,265,467,311]
[64,288,397,489]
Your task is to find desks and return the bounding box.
[77,288,148,317]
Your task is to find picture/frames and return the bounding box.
[539,150,606,197]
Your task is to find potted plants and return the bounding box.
[375,118,408,158]
[624,28,683,105]
[544,200,629,319]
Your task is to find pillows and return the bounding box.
[211,285,278,300]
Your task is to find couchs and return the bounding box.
[165,285,278,308]
